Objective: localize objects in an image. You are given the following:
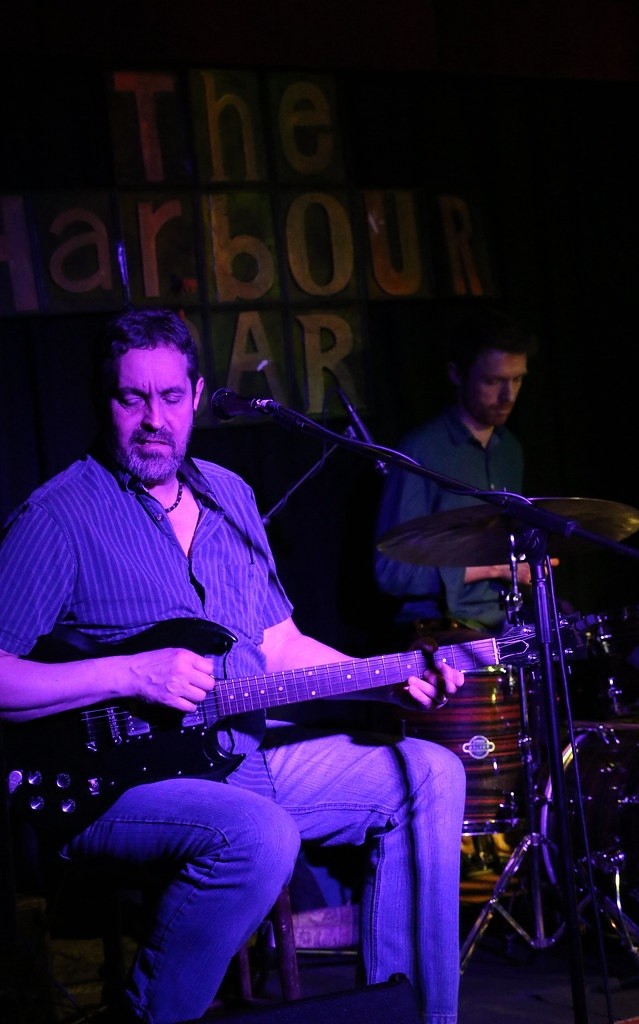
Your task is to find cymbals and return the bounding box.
[373,493,639,572]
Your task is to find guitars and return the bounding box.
[0,610,587,848]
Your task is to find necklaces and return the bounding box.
[164,478,183,513]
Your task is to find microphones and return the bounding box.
[339,392,392,483]
[210,387,283,420]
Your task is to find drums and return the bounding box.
[392,609,536,906]
[560,718,639,820]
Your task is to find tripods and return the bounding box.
[456,528,639,979]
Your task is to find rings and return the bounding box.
[441,696,447,702]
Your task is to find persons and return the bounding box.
[375,328,597,664]
[0,309,467,1024]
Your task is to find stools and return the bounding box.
[71,873,300,1024]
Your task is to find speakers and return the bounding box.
[215,973,425,1024]
